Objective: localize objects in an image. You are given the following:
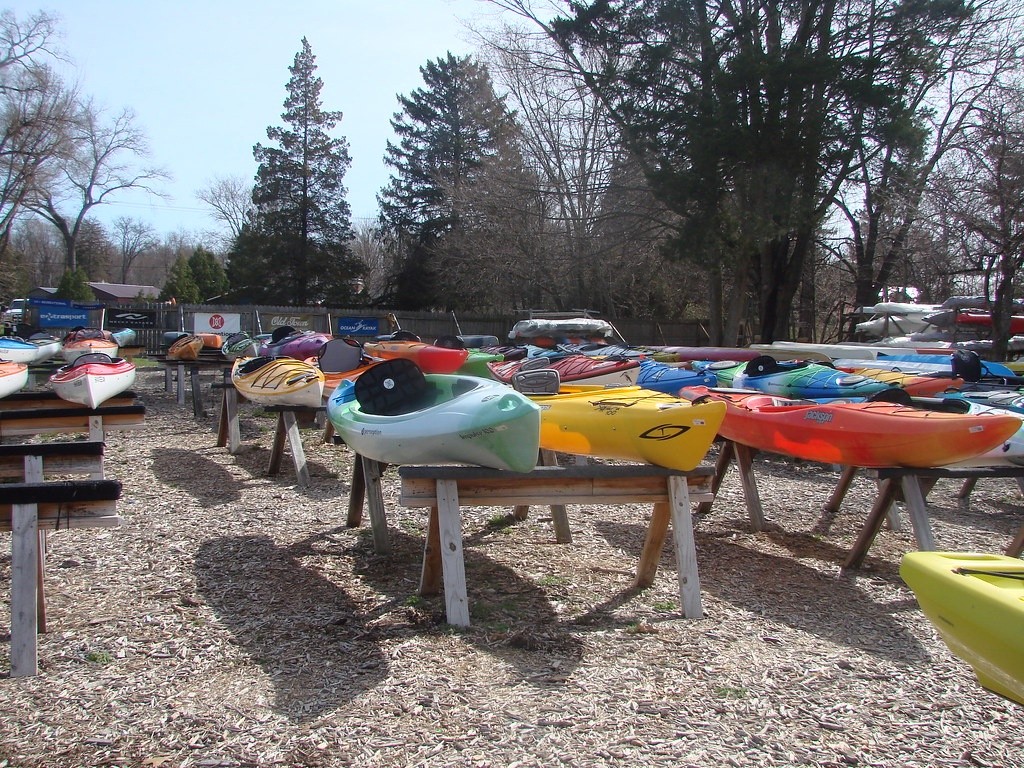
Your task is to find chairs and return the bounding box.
[273,326,296,342]
[318,338,371,371]
[74,353,113,367]
[951,348,1008,386]
[434,335,465,349]
[867,387,916,408]
[389,329,422,343]
[76,328,105,340]
[28,329,50,339]
[227,333,247,349]
[746,355,778,378]
[513,368,561,397]
[352,357,439,416]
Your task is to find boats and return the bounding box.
[222,327,383,398]
[165,332,223,349]
[900,552,1024,704]
[62,328,119,364]
[679,385,1022,468]
[168,335,204,360]
[505,369,727,472]
[362,296,1024,456]
[231,356,325,408]
[0,335,60,399]
[327,359,540,473]
[45,353,136,410]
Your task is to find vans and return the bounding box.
[2,299,66,336]
